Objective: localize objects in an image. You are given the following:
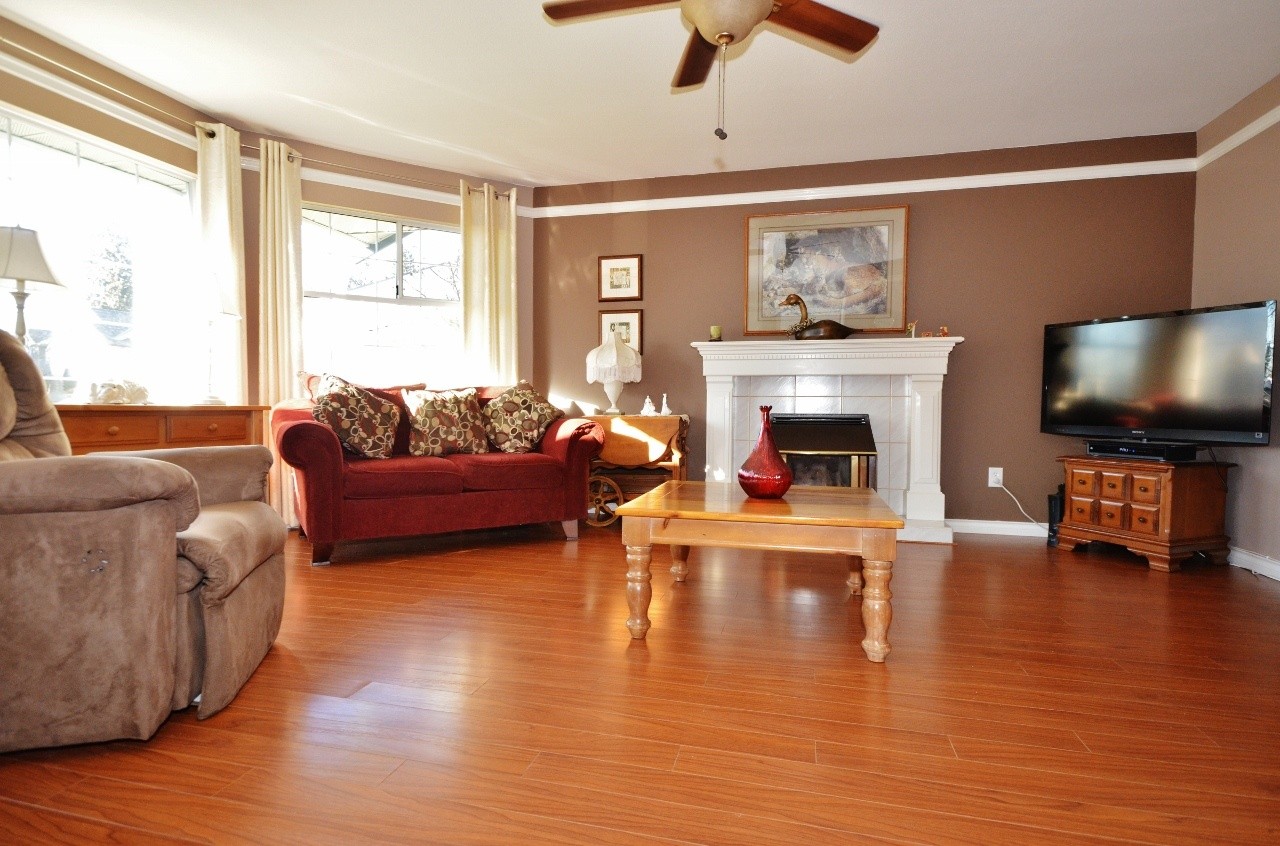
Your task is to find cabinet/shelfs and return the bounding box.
[52,405,272,455]
[1053,455,1238,572]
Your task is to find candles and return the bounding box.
[710,326,721,338]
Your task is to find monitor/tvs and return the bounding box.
[1039,298,1276,447]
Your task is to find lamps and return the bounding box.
[586,331,641,416]
[682,0,775,140]
[0,226,60,347]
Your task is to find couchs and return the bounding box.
[0,331,289,754]
[273,398,605,567]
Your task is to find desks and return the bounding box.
[615,480,904,662]
[569,414,690,528]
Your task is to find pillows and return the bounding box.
[312,374,400,460]
[480,381,565,456]
[399,388,490,456]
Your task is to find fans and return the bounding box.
[544,0,881,92]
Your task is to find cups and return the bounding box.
[708,325,722,341]
[939,327,948,337]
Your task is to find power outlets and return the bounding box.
[989,466,1003,488]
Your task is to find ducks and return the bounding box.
[778,295,864,341]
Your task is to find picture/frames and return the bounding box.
[597,309,643,355]
[598,253,643,302]
[742,203,910,336]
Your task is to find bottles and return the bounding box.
[738,405,792,499]
